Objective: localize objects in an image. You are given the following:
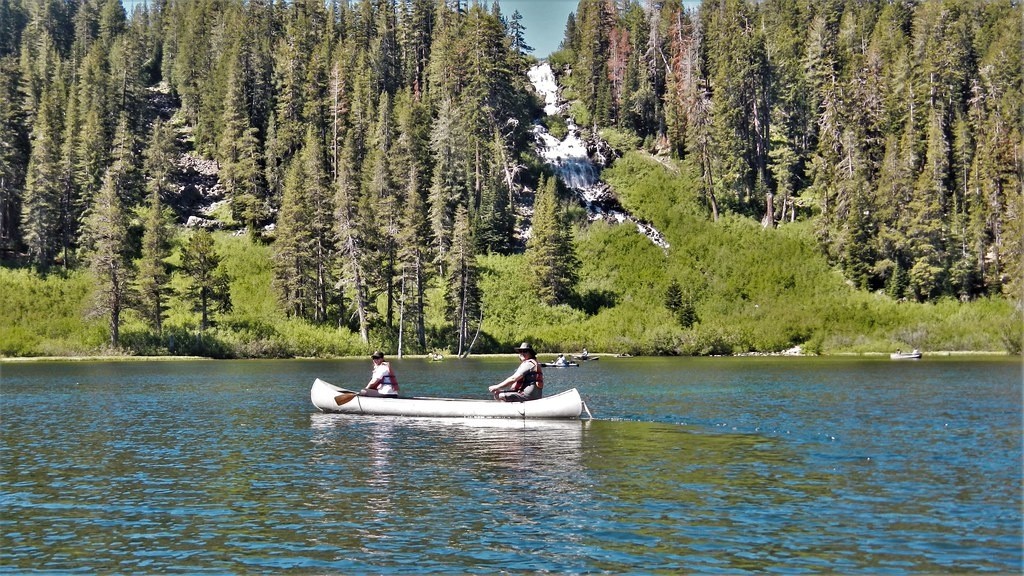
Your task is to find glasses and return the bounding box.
[372,357,382,360]
[519,350,531,354]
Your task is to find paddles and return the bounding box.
[334,391,360,406]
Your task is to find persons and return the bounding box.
[364,351,399,399]
[488,342,590,402]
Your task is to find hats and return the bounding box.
[558,353,563,355]
[371,351,384,358]
[514,342,537,355]
[583,348,587,351]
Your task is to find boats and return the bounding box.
[889,349,922,359]
[614,353,633,358]
[309,378,584,419]
[537,358,580,367]
[569,355,600,362]
